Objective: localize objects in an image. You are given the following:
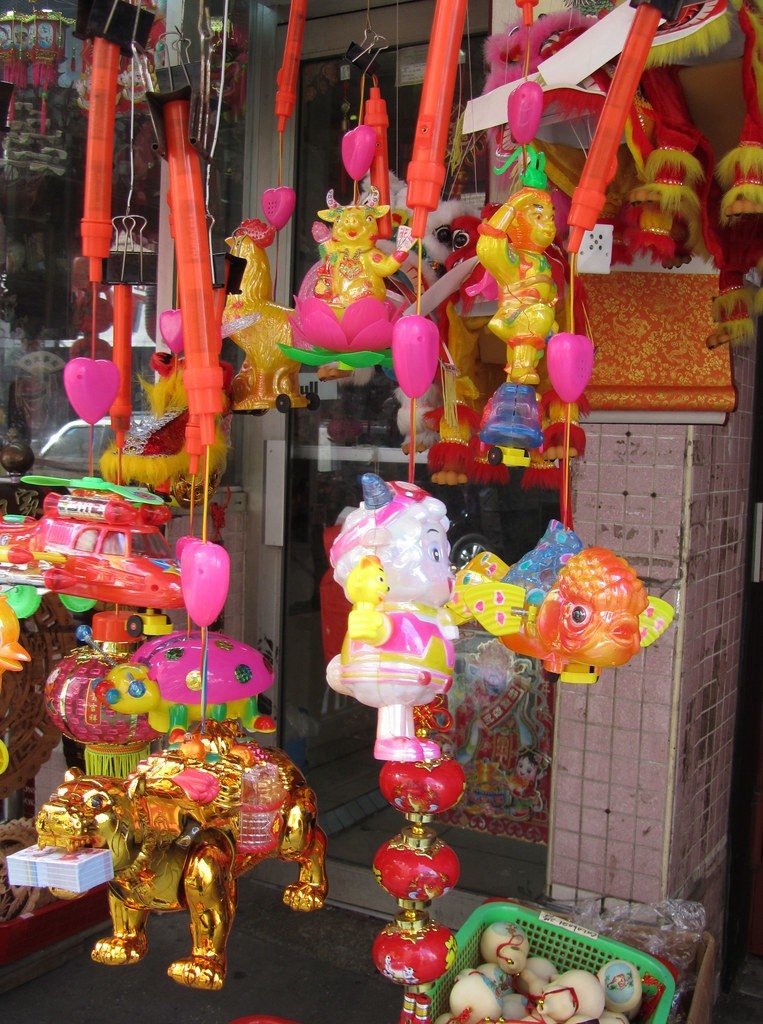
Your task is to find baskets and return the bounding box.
[403,902,675,1024]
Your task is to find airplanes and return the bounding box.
[0,474,186,611]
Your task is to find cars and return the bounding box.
[40,415,151,469]
